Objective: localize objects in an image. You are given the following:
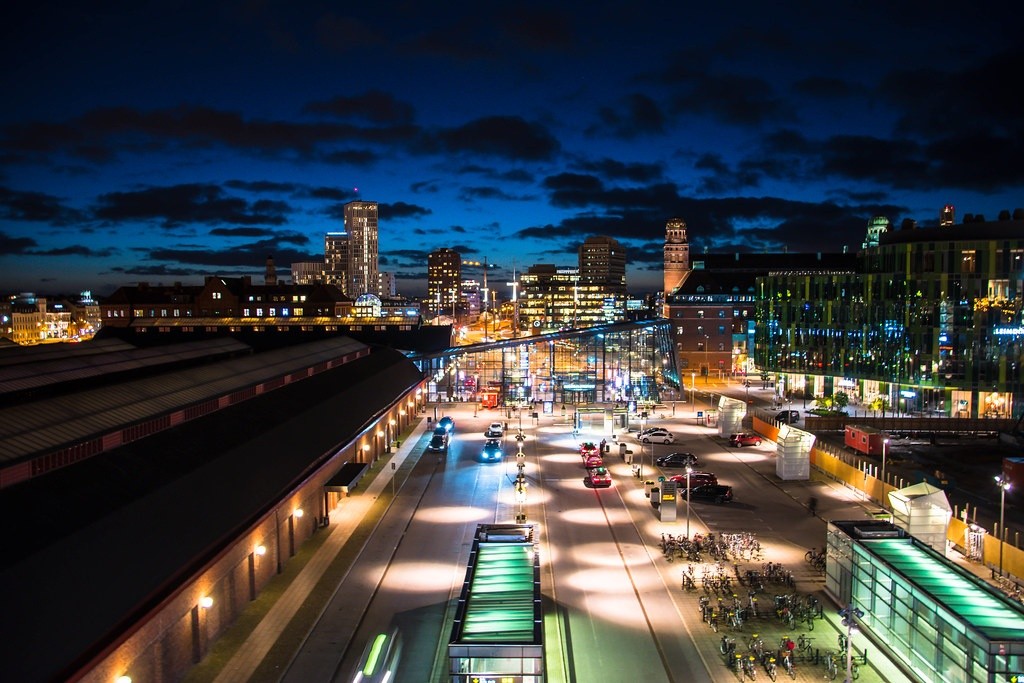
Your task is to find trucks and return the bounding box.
[1003,456,1024,494]
[844,423,889,456]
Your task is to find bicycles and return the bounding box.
[839,603,856,683]
[718,635,737,665]
[823,634,860,679]
[745,656,756,680]
[780,636,795,661]
[764,651,777,682]
[796,635,815,662]
[747,633,763,659]
[732,654,745,682]
[657,526,827,631]
[778,650,797,680]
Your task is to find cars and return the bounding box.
[578,441,612,488]
[425,416,455,454]
[776,408,800,425]
[729,431,762,449]
[637,425,734,504]
[479,423,504,462]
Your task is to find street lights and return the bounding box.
[703,334,709,363]
[881,436,890,509]
[510,423,531,525]
[993,468,1012,575]
[685,467,693,540]
